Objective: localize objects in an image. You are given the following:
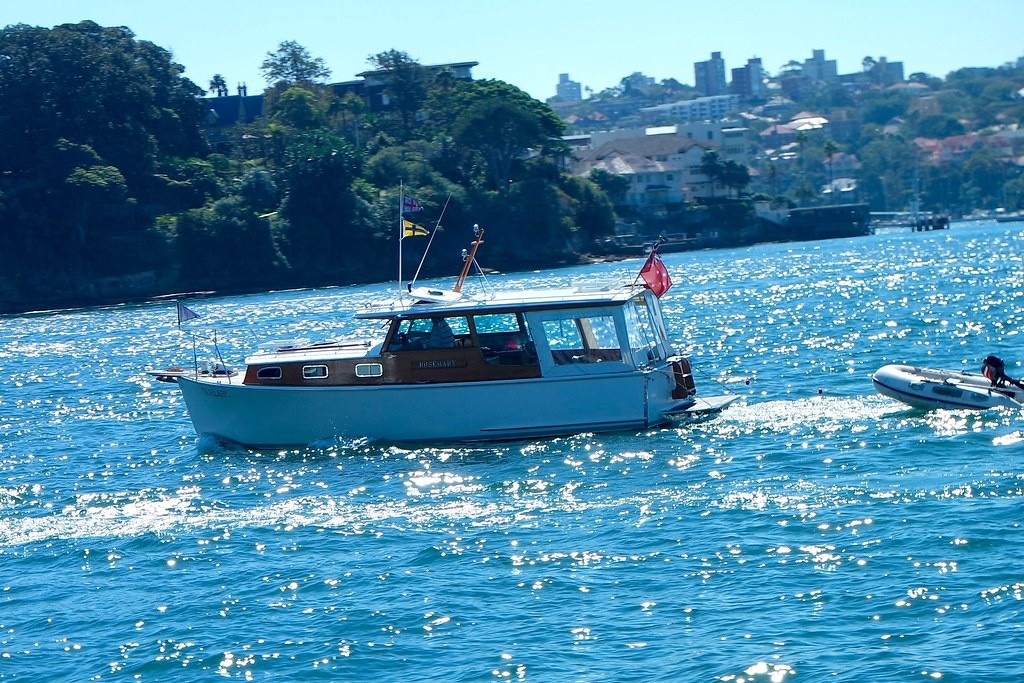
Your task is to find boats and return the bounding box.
[146,177,746,455]
[871,364,1024,412]
[962,207,1024,223]
